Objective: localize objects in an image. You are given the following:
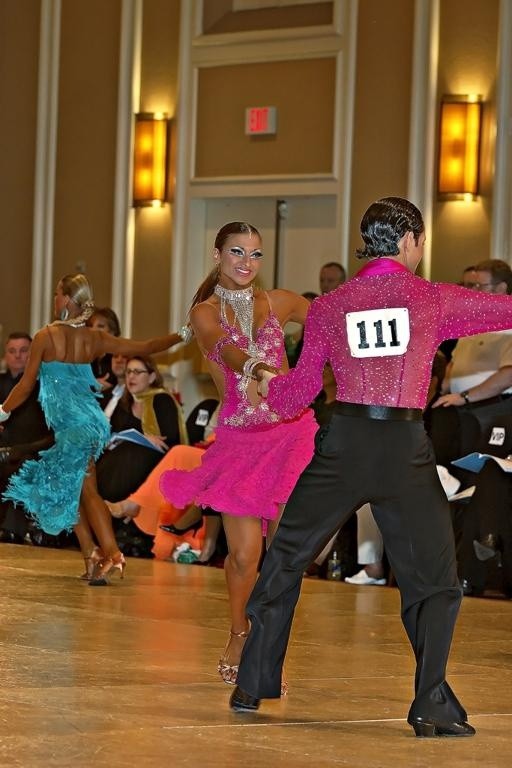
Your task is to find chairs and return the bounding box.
[186,398,218,446]
[1,433,55,546]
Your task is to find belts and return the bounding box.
[332,399,423,422]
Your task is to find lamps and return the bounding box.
[437,94,481,206]
[130,110,169,209]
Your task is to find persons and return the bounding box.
[229,197,512,738]
[159,222,310,695]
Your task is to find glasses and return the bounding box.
[126,368,149,375]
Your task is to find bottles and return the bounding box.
[326,541,342,581]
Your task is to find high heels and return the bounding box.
[218,628,250,685]
[88,550,126,586]
[80,546,105,581]
[191,545,218,566]
[159,515,203,538]
[104,500,140,525]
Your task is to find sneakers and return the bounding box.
[345,569,387,585]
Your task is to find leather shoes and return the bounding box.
[228,686,262,714]
[410,716,476,739]
